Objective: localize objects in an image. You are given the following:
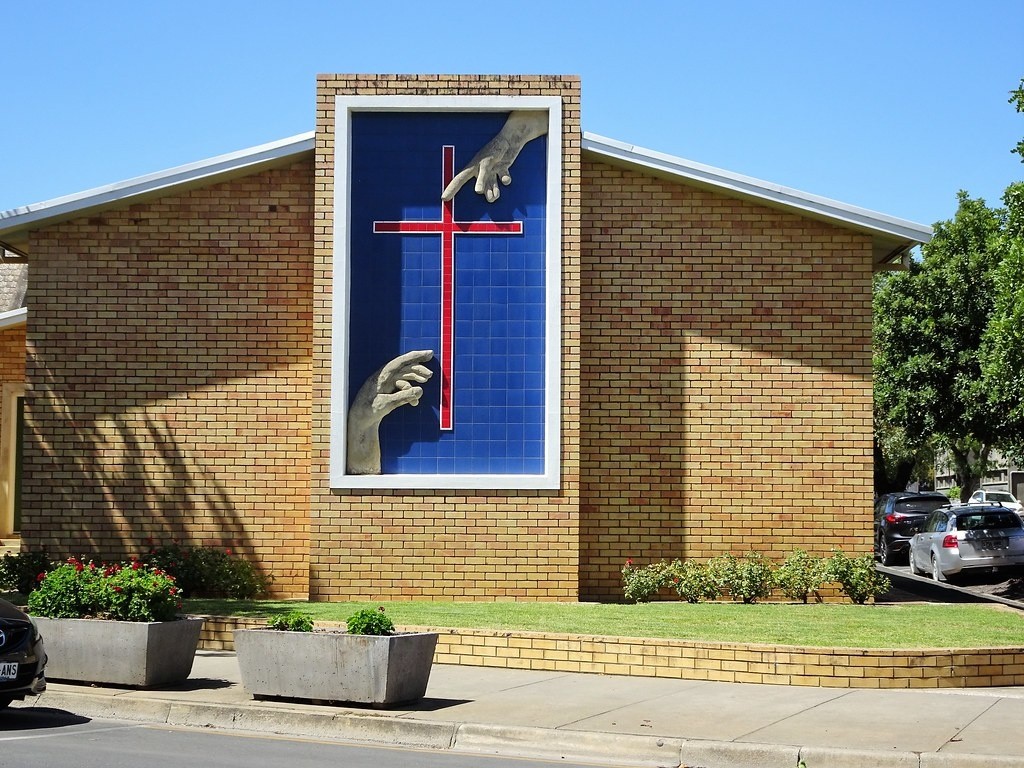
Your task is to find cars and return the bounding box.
[0,597,50,714]
[907,501,1024,582]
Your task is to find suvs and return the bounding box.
[873,490,953,567]
[967,488,1024,521]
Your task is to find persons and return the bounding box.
[346,110,549,475]
